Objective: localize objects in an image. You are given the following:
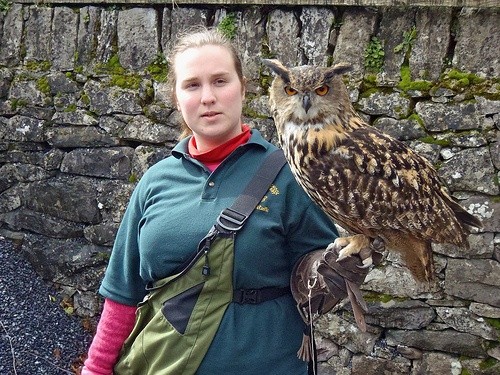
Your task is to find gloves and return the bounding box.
[290,247,370,361]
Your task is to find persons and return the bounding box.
[81,25,384,375]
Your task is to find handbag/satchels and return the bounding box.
[113,225,235,375]
